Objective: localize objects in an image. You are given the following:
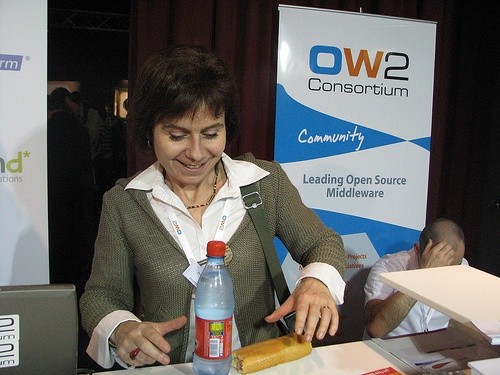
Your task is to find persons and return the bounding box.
[47,42,347,368]
[362,218,469,341]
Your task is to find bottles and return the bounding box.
[193,241,233,375]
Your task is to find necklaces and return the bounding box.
[186,172,218,209]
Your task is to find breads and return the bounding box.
[230,332,312,374]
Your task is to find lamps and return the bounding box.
[112,70,129,178]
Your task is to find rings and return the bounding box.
[321,306,332,309]
[129,348,141,360]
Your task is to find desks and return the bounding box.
[89,327,500,375]
